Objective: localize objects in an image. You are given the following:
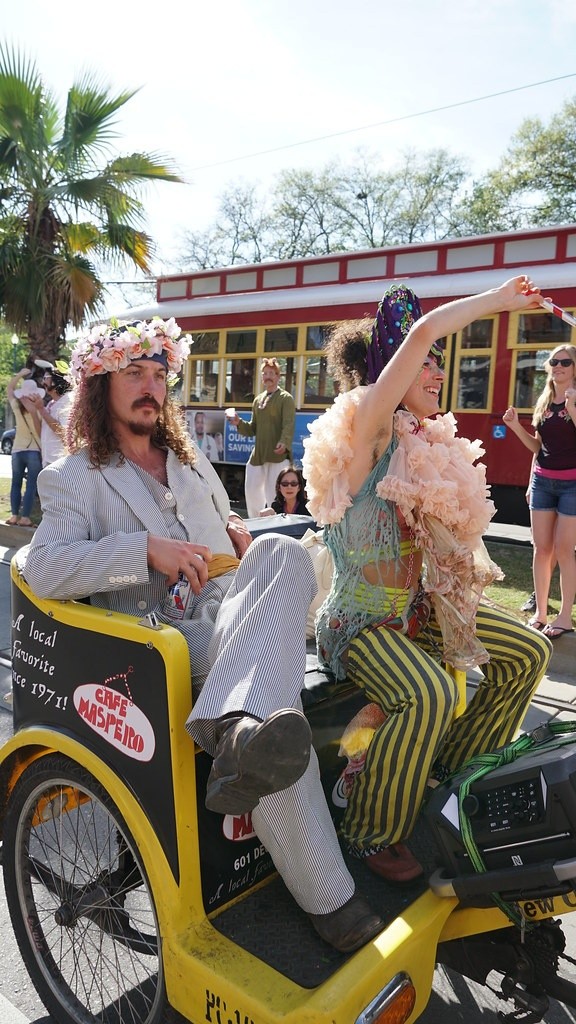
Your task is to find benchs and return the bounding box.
[187,627,365,756]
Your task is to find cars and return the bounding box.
[1,426,17,455]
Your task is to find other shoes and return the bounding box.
[363,841,424,887]
[522,591,538,613]
[6,518,31,526]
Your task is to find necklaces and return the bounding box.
[258,386,280,410]
[46,393,65,415]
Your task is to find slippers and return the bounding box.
[526,621,546,630]
[541,624,574,638]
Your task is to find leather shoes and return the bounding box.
[205,708,312,815]
[309,894,384,952]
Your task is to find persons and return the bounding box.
[5,369,41,526]
[291,371,315,395]
[190,412,219,462]
[226,358,296,518]
[260,468,311,516]
[28,321,384,954]
[520,453,557,612]
[214,433,224,462]
[303,275,551,885]
[20,367,73,468]
[504,344,575,640]
[206,373,229,403]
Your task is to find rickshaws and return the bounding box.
[0,516,576,1024]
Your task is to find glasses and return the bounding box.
[279,481,300,486]
[549,358,574,366]
[42,375,52,381]
[262,371,279,376]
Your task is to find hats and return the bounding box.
[14,379,46,400]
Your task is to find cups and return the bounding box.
[225,408,237,422]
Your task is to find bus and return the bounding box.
[90,222,576,529]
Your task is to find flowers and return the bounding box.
[68,314,195,385]
